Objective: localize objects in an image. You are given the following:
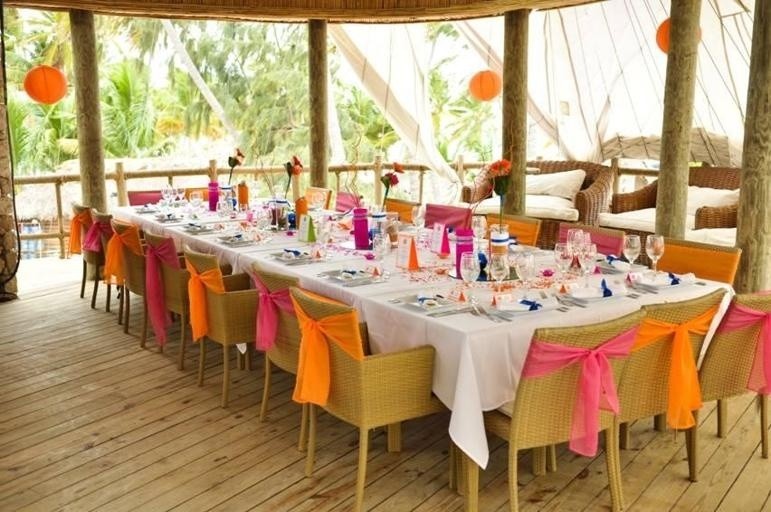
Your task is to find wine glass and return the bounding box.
[163,182,665,302]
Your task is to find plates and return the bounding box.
[399,277,707,323]
[134,205,186,224]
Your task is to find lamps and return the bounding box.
[654,17,705,56]
[24,64,68,105]
[468,70,502,101]
